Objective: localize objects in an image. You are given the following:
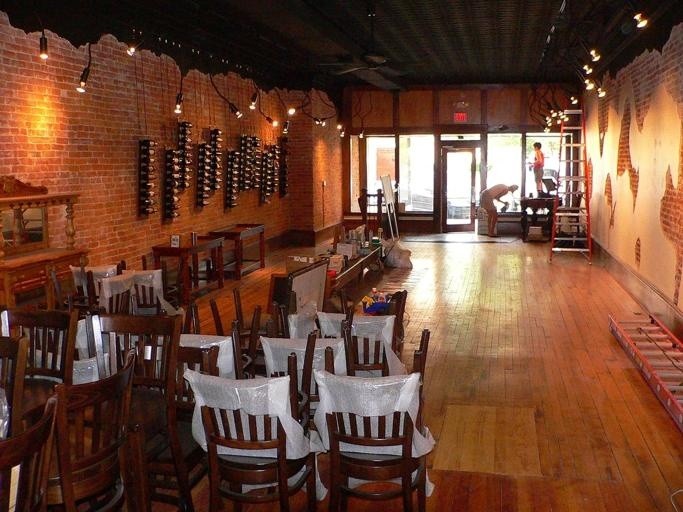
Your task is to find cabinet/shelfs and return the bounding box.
[0,174,91,309]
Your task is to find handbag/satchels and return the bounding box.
[384,247,412,269]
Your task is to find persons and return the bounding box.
[481,183,518,237]
[528,142,544,194]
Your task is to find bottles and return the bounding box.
[140,121,291,218]
[371,287,385,303]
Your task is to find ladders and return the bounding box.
[607,313,683,434]
[548,94,593,265]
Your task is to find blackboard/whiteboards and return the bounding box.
[380,174,393,204]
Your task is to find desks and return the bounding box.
[151,233,226,303]
[318,243,384,312]
[210,222,265,279]
[520,197,557,242]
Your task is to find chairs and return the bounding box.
[1,263,430,510]
[558,192,583,235]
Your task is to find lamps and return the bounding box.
[336,78,362,130]
[301,91,320,125]
[529,0,648,132]
[359,89,372,137]
[40,28,48,59]
[209,75,243,119]
[126,39,145,56]
[316,91,339,128]
[289,90,311,115]
[77,44,94,92]
[173,76,184,114]
[252,81,278,127]
[339,88,362,137]
[274,88,290,134]
[249,87,260,111]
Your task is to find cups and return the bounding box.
[191,231,198,241]
